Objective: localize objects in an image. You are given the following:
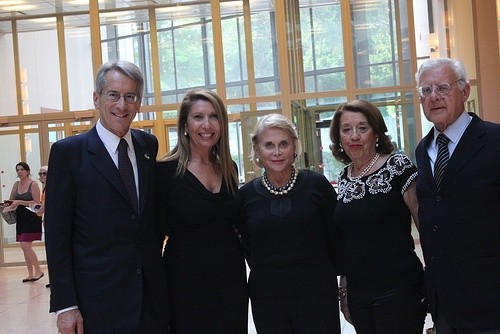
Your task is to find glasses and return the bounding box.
[418,79,463,97]
[38,172,47,176]
[101,92,139,103]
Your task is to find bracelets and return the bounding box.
[338,286,347,301]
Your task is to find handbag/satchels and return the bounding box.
[0,203,17,225]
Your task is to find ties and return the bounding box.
[434,133,451,193]
[117,138,139,215]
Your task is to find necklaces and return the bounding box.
[262,166,298,195]
[347,153,380,181]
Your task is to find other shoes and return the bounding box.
[30,273,44,281]
[22,278,31,283]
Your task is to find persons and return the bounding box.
[29,166,50,287]
[140,86,249,334]
[329,99,426,334]
[238,111,340,334]
[4,162,44,282]
[44,60,159,334]
[415,57,500,334]
